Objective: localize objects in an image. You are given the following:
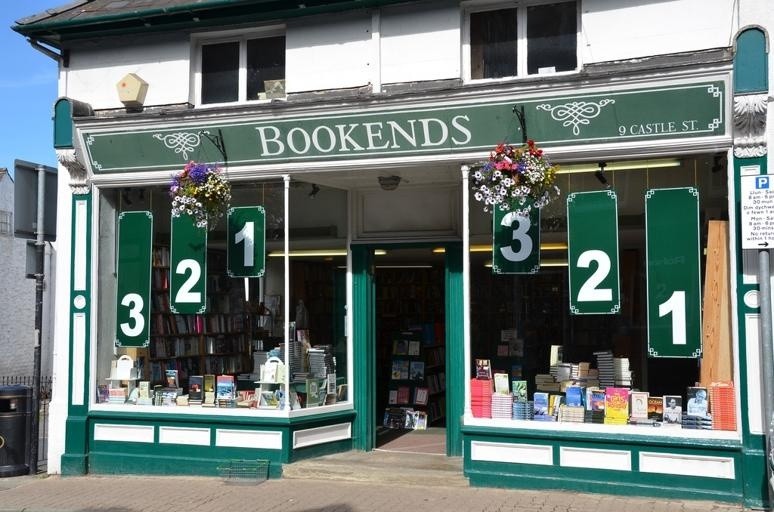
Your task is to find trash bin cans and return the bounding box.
[0,383,33,478]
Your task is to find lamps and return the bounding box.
[485,260,569,268]
[432,244,568,254]
[309,183,320,200]
[550,157,681,176]
[594,163,613,190]
[267,249,387,257]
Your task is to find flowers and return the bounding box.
[468,139,562,221]
[169,160,236,232]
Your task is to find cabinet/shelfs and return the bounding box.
[146,243,259,395]
[382,321,446,432]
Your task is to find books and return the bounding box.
[97,369,255,408]
[248,323,336,381]
[534,344,637,392]
[384,327,448,430]
[491,327,524,379]
[151,250,250,385]
[470,357,736,430]
